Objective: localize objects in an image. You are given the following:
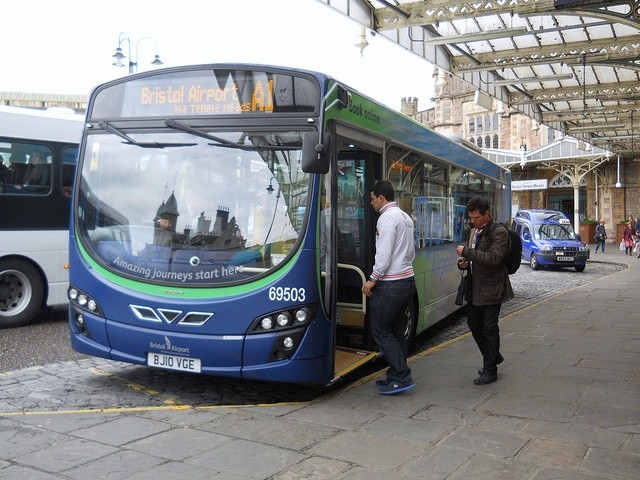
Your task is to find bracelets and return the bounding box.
[369,277,377,283]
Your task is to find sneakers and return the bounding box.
[377,378,416,396]
[374,377,396,389]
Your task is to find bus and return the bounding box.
[0,106,87,324]
[66,62,513,402]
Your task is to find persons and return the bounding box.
[456,195,514,385]
[622,220,635,256]
[0,156,10,185]
[633,218,640,237]
[62,164,72,186]
[360,179,417,394]
[26,149,50,187]
[594,220,607,254]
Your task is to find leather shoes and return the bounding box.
[473,365,498,385]
[478,353,504,375]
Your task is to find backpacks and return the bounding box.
[488,222,522,275]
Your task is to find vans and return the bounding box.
[512,208,591,272]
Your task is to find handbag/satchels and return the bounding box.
[594,232,599,240]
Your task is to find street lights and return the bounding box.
[112,31,164,75]
